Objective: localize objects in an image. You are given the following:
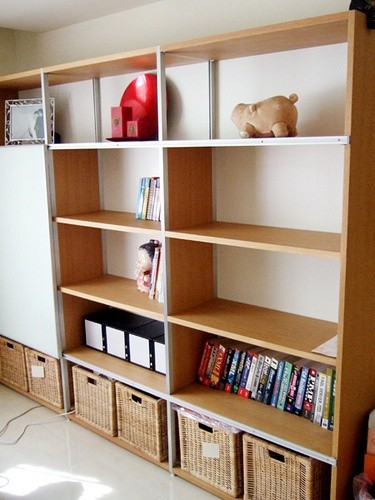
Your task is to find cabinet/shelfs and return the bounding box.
[0,9,375,500]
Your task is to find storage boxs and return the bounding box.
[241,432,327,500]
[177,409,241,500]
[114,381,168,463]
[0,336,29,394]
[23,346,64,412]
[70,366,118,438]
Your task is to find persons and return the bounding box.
[14,108,44,139]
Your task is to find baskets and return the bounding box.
[1,335,29,394]
[25,348,63,409]
[177,408,244,497]
[240,434,324,500]
[72,365,117,439]
[114,380,169,463]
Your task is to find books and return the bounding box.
[197,337,336,432]
[147,247,164,304]
[132,176,162,222]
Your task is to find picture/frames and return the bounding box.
[4,96,56,145]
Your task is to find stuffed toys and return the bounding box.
[230,93,298,138]
